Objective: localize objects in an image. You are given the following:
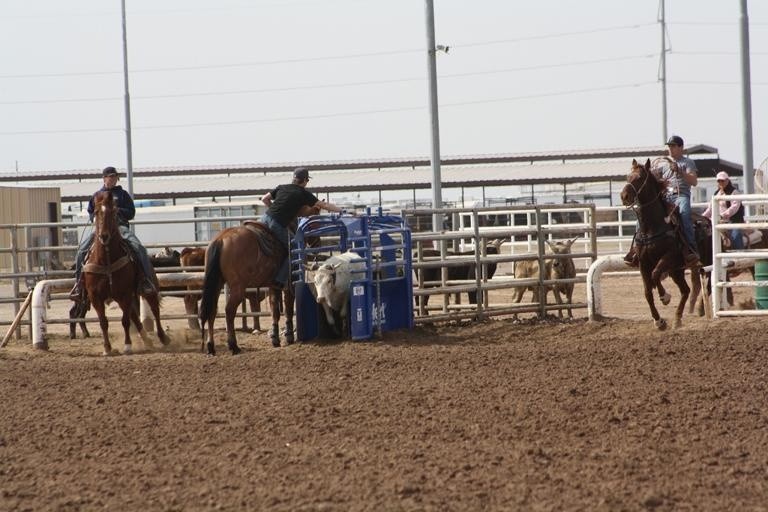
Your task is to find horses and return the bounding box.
[620,157,723,332]
[688,221,768,317]
[198,199,326,358]
[84,190,171,357]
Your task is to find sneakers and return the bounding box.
[144,279,152,294]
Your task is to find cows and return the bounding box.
[413,237,506,325]
[179,246,270,333]
[68,248,179,339]
[301,252,367,340]
[511,235,581,326]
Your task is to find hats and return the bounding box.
[103,167,116,176]
[294,169,311,178]
[665,136,683,145]
[716,171,729,180]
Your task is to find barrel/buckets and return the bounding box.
[754,259,768,310]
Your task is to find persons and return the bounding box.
[623,135,702,270]
[255,166,358,291]
[702,171,746,250]
[68,166,159,311]
